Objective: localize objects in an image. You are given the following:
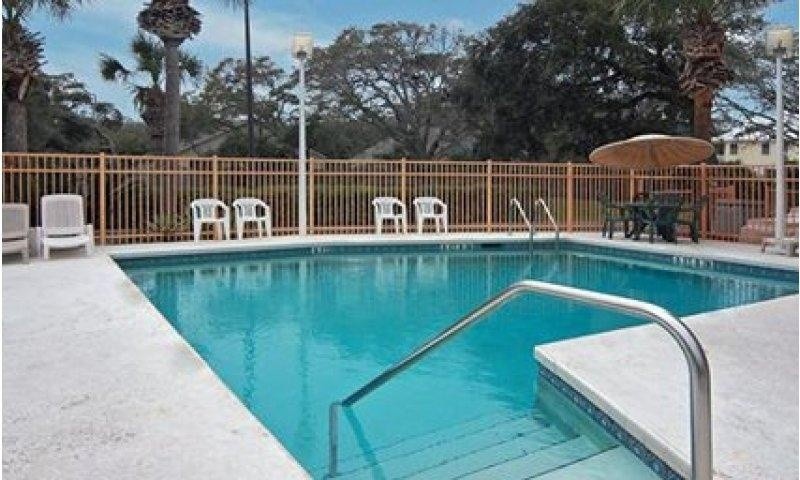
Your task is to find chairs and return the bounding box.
[35,195,95,260]
[2,204,29,264]
[189,199,230,242]
[371,197,407,235]
[233,199,271,240]
[596,191,699,242]
[413,197,447,234]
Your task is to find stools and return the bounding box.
[759,236,799,258]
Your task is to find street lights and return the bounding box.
[291,29,317,238]
[762,27,798,260]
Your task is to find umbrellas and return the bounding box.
[589,133,716,217]
